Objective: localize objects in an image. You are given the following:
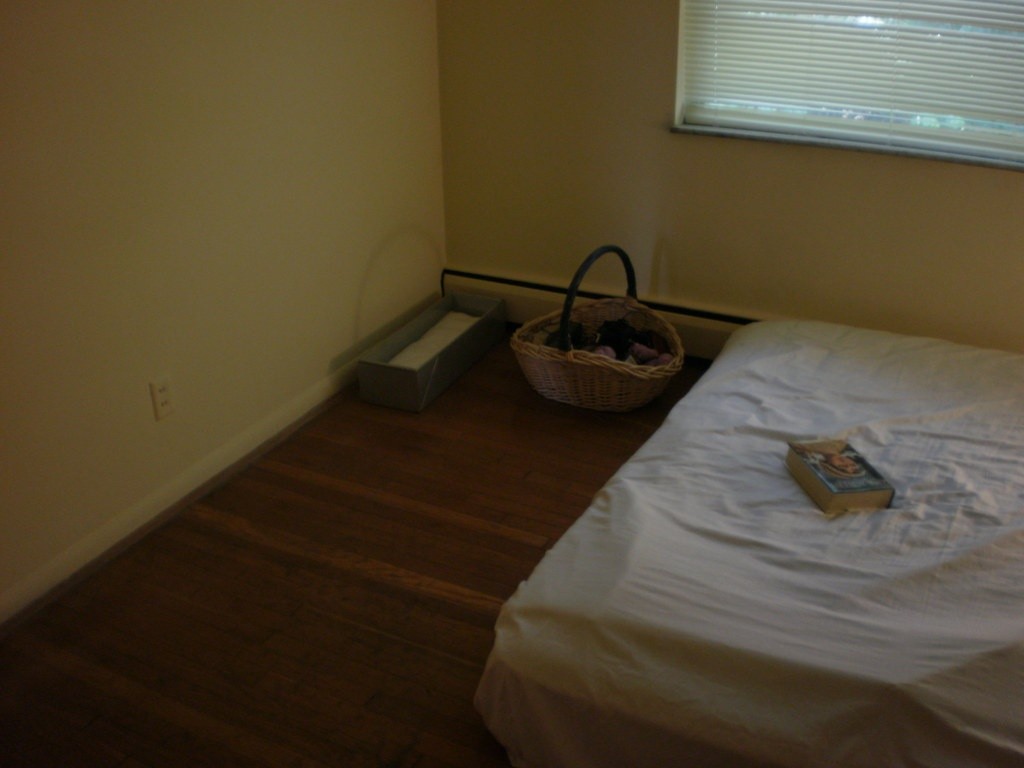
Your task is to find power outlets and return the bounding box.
[150,376,176,418]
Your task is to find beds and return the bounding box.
[473,320,1024,768]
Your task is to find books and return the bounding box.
[784,440,895,514]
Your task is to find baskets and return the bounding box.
[510,244,684,411]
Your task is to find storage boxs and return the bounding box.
[359,292,504,412]
